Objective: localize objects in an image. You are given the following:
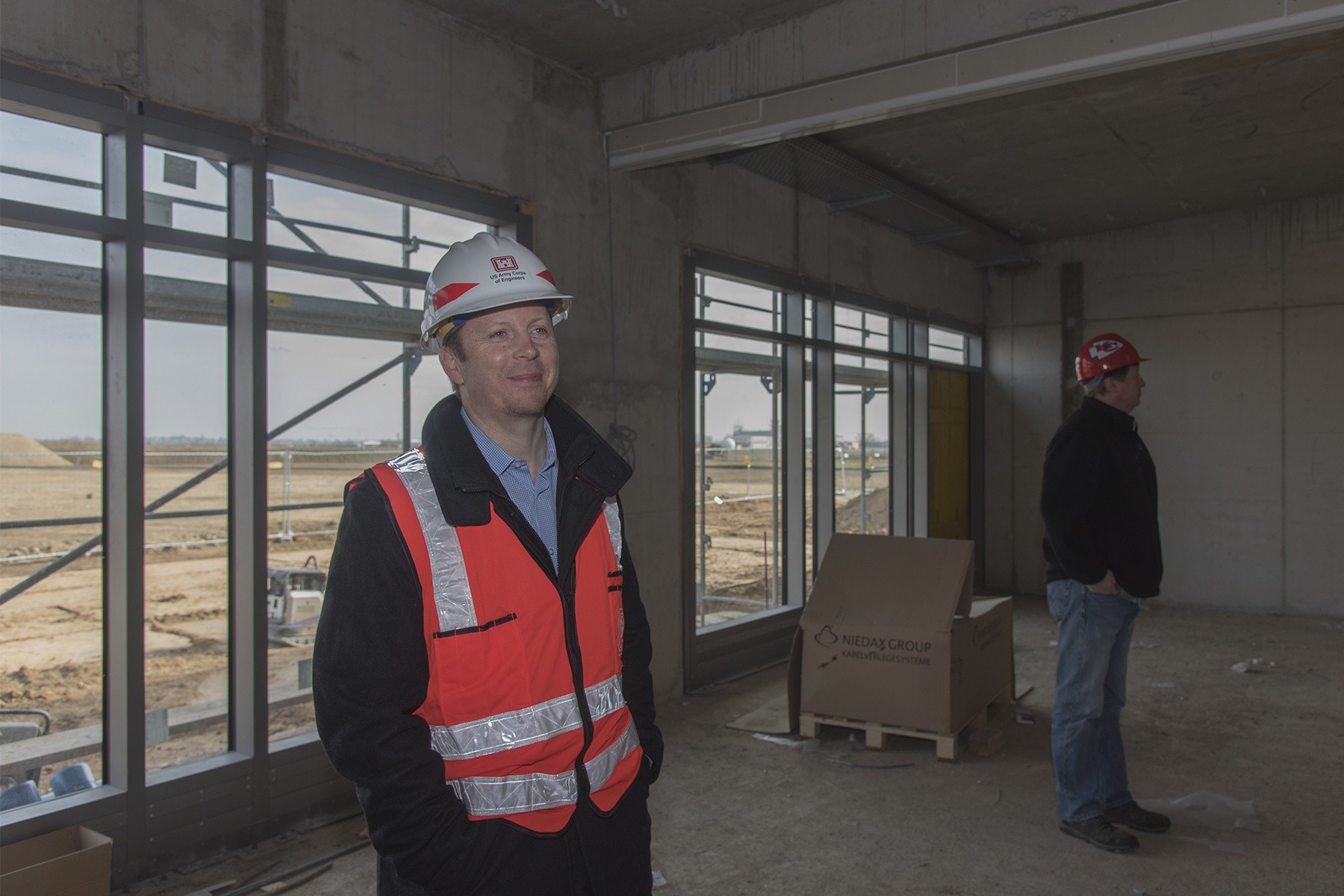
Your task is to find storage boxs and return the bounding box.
[0,825,113,896]
[800,531,1013,734]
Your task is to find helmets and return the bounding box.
[420,232,574,356]
[1075,332,1152,382]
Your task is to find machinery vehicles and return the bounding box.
[267,555,327,647]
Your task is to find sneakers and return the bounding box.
[1059,815,1138,851]
[1104,800,1170,832]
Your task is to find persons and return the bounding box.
[1037,334,1173,851]
[315,230,664,896]
